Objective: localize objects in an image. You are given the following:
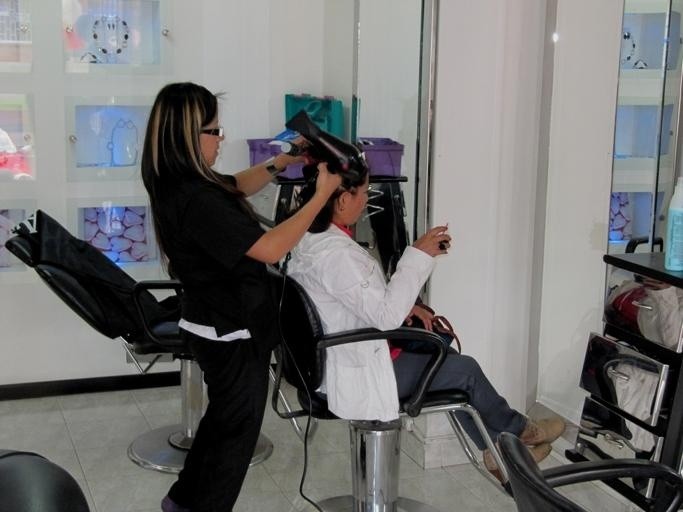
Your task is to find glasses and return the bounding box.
[200,127,223,138]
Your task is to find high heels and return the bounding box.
[482,443,553,482]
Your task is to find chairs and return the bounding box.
[9,209,272,473]
[269,267,518,512]
[498,431,682,510]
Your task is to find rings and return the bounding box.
[440,242,450,249]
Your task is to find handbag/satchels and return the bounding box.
[402,302,462,355]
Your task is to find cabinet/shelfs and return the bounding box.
[564,250,683,512]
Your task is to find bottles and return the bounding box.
[666,176,683,271]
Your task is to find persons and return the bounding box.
[142,83,342,512]
[278,160,565,471]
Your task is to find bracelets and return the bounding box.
[265,157,286,176]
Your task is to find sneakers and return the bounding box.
[519,419,566,446]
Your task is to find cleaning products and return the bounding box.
[665,176,683,273]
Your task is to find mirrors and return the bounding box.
[609,0,683,256]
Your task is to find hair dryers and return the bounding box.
[285,110,369,210]
